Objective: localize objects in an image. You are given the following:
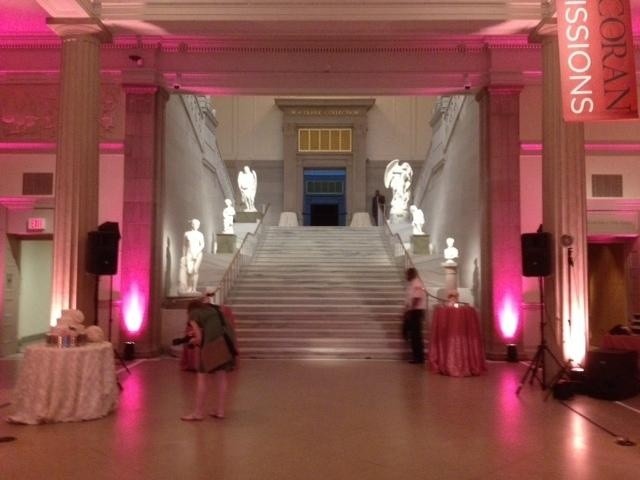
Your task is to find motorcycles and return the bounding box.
[515,278,571,394]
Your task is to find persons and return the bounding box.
[443,237,459,262]
[237,166,257,212]
[181,217,205,291]
[384,158,413,213]
[401,267,428,364]
[222,198,237,234]
[181,296,239,421]
[372,189,386,225]
[410,205,425,236]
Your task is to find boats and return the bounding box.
[520,232,552,277]
[87,231,118,275]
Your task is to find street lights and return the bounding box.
[200,335,236,373]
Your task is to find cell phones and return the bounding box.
[173,334,193,345]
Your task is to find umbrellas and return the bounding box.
[408,359,425,363]
[209,409,225,419]
[179,413,203,420]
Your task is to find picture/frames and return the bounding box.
[26,341,115,423]
[430,303,485,377]
